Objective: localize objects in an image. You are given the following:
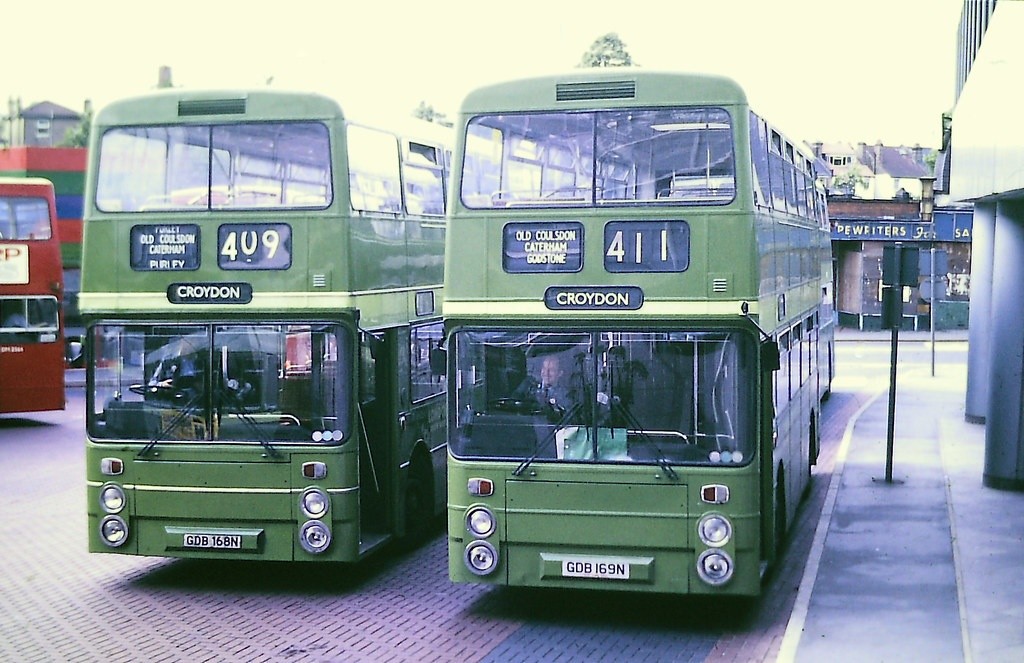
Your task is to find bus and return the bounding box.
[426,65,836,597]
[1,175,67,417]
[65,86,501,564]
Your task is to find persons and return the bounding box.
[151,334,205,404]
[512,355,578,425]
[1,304,50,334]
